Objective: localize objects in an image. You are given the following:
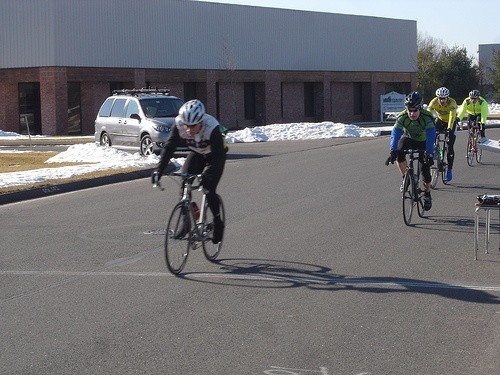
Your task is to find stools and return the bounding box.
[473,202,500,260]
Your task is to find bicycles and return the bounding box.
[386,148,429,226]
[429,128,450,188]
[456,115,482,166]
[151,163,226,274]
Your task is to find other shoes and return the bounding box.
[399,174,410,192]
[212,214,224,243]
[445,168,452,180]
[174,211,190,239]
[424,193,431,210]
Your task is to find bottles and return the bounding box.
[439,147,443,160]
[415,174,418,189]
[474,136,477,145]
[192,202,200,220]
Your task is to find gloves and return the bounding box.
[424,152,434,166]
[151,170,161,183]
[384,150,397,166]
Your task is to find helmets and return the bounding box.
[403,90,423,111]
[178,98,205,126]
[468,89,480,98]
[435,86,450,98]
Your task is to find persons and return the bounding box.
[384,91,436,210]
[426,88,458,181]
[152,99,229,244]
[457,90,489,157]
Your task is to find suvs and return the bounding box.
[94,89,185,158]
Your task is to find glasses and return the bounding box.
[409,109,418,112]
